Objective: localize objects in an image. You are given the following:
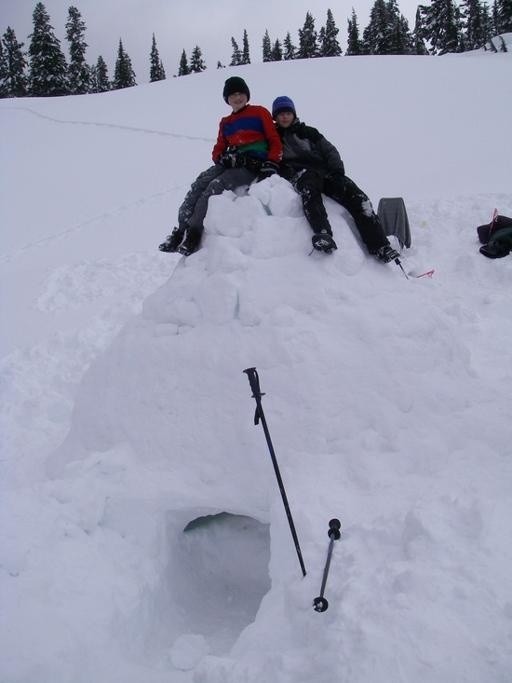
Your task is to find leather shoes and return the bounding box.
[280,164,295,177]
[217,151,237,168]
[325,181,335,196]
[257,160,279,182]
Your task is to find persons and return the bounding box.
[155,73,284,256]
[268,93,401,266]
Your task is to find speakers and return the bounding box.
[158,225,183,252]
[179,226,204,252]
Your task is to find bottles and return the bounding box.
[272,95,297,120]
[222,76,250,107]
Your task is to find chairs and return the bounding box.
[377,247,398,263]
[311,233,337,254]
